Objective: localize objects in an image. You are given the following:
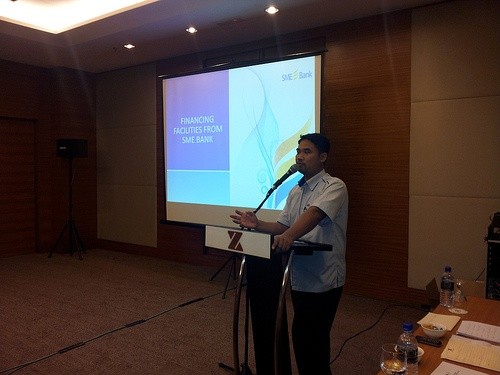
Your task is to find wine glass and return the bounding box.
[447,279,469,314]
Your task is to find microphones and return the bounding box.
[266,163,300,196]
[484,240,500,302]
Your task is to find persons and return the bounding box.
[229,133,349,375]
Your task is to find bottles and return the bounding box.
[440,266,454,307]
[396,323,418,375]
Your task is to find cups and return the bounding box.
[379,343,407,375]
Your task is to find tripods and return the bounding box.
[47,160,87,261]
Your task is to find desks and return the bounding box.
[376,295,500,375]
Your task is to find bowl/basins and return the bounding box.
[394,344,424,362]
[421,322,446,339]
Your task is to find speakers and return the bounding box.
[56,138,90,158]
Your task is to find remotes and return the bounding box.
[415,336,442,347]
